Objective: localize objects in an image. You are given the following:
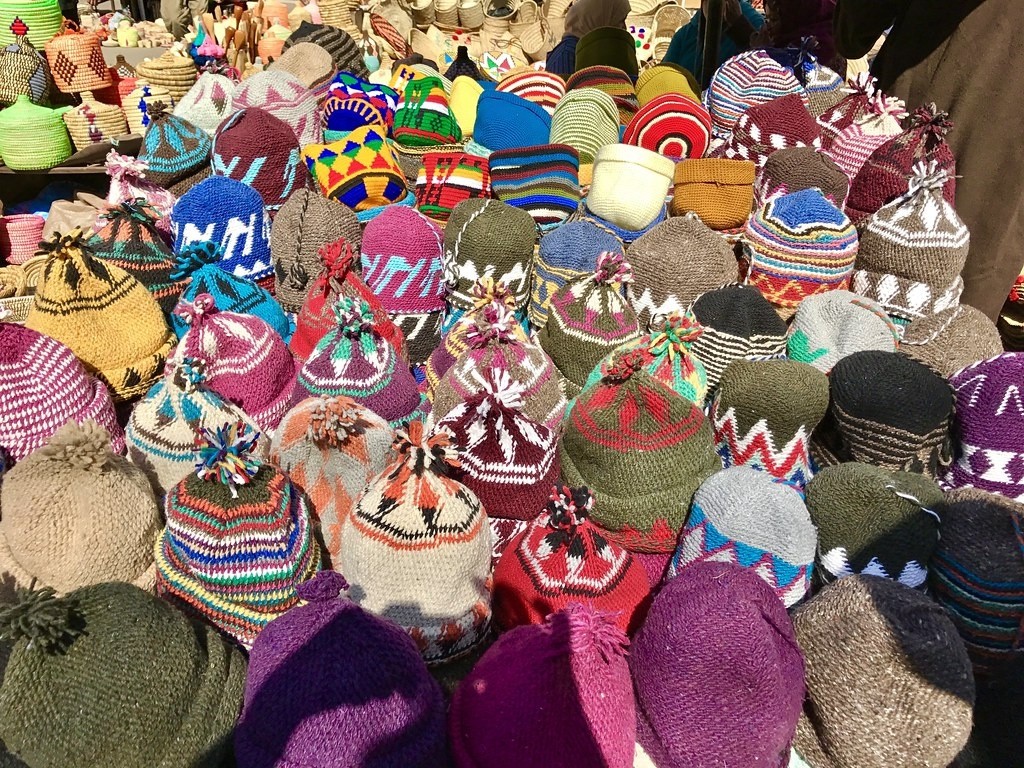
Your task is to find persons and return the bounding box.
[545,0,639,86]
[160,0,209,41]
[657,0,765,91]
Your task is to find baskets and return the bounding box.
[411,0,695,67]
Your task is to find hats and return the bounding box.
[0,16,1022,766]
